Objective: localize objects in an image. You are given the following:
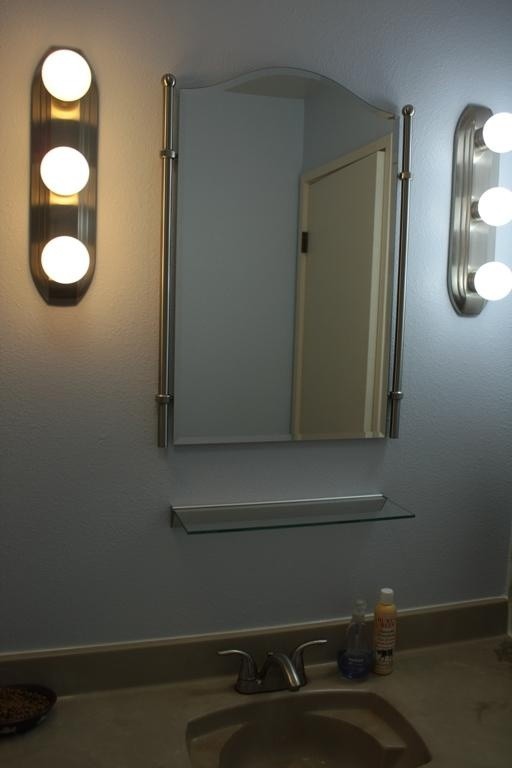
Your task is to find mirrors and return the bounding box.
[153,62,419,450]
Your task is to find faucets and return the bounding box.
[261,651,301,690]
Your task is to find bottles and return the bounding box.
[336,599,375,680]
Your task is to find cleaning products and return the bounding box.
[338,600,370,680]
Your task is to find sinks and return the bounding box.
[186,689,430,768]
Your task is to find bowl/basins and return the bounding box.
[0,684,58,736]
